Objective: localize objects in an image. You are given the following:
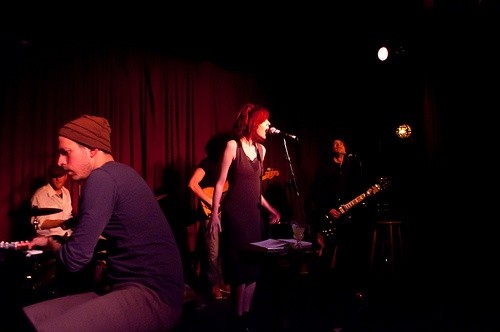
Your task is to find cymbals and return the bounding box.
[7,206,63,218]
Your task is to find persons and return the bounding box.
[206,103,284,332]
[28,161,77,237]
[20,112,185,332]
[187,129,240,300]
[309,136,380,294]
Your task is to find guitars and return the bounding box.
[192,168,279,219]
[315,176,393,239]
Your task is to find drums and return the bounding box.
[17,245,50,270]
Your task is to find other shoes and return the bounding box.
[220,283,230,293]
[209,287,222,299]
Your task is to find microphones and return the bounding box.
[348,154,360,160]
[269,127,299,142]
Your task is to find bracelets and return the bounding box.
[211,210,222,217]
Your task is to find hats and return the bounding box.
[57,114,111,154]
[49,167,65,178]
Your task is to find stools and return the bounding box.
[371,219,407,275]
